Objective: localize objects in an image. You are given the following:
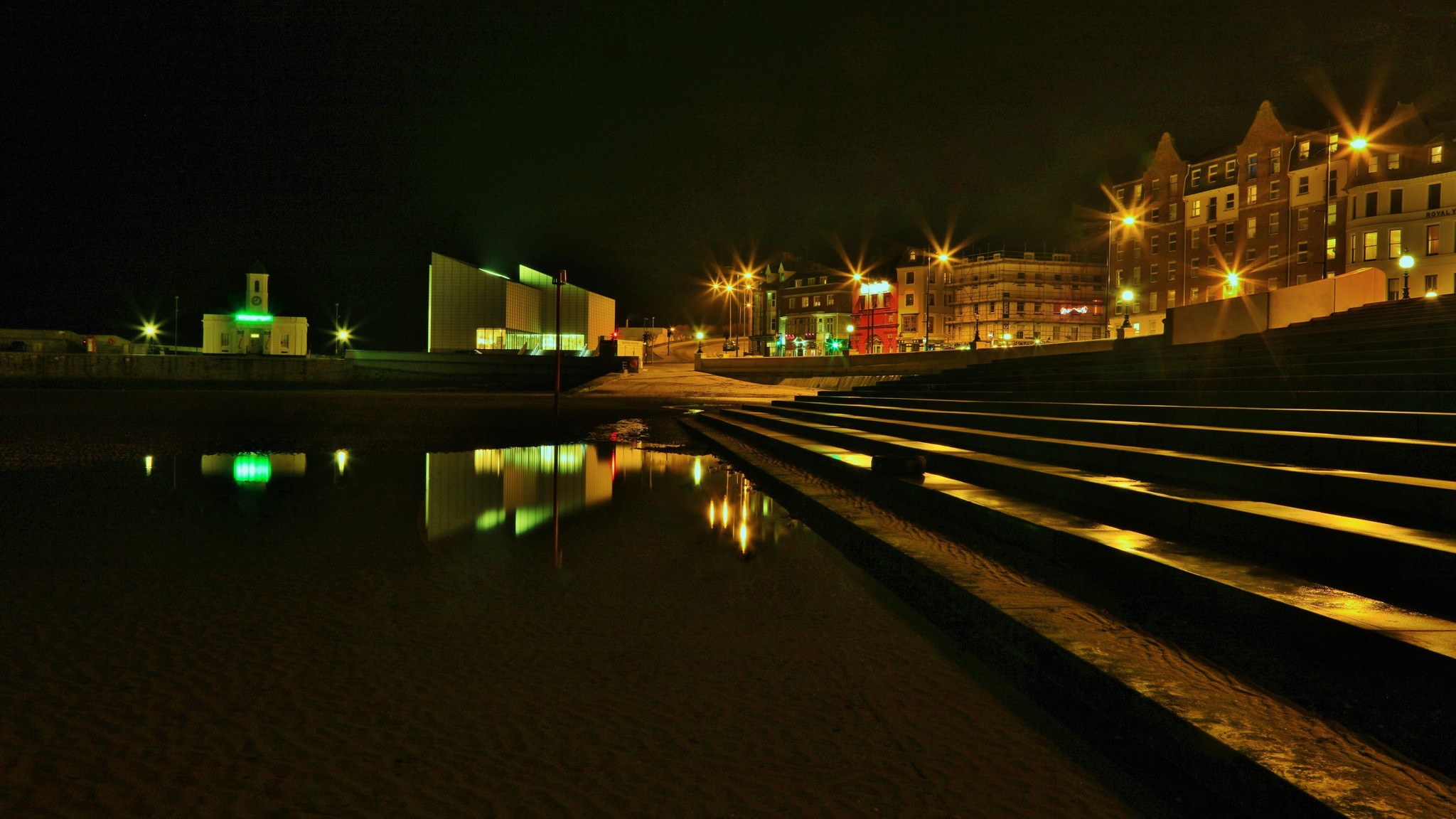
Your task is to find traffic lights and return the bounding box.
[642,334,646,342]
[723,331,729,339]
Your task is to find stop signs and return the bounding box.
[922,338,927,344]
[988,332,993,339]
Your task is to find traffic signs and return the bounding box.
[898,334,903,341]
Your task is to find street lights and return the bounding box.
[851,268,870,354]
[552,270,567,398]
[1399,247,1414,300]
[549,445,563,571]
[871,301,878,353]
[1314,133,1366,280]
[744,285,751,352]
[972,311,982,342]
[340,333,346,359]
[847,326,854,349]
[1100,216,1135,338]
[1120,291,1133,328]
[727,286,732,342]
[927,245,947,351]
[147,327,154,355]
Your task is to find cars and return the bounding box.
[723,341,739,351]
